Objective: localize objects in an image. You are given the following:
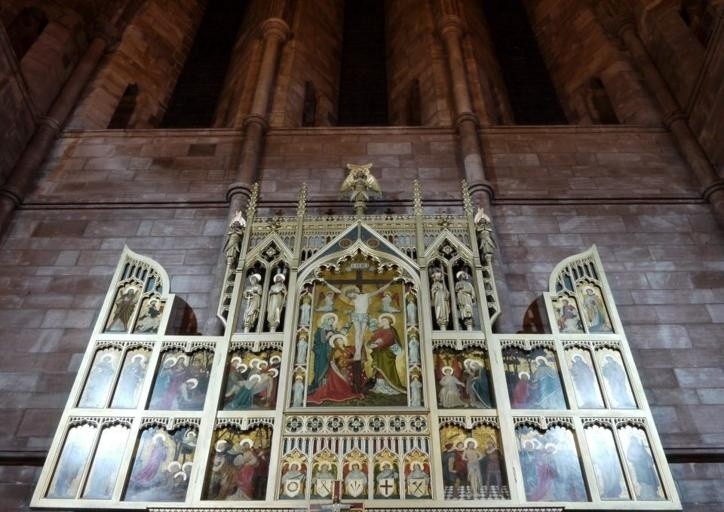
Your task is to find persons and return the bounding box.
[474,206,495,255]
[406,297,417,326]
[557,297,583,334]
[350,169,371,207]
[314,269,404,363]
[306,334,364,405]
[454,271,475,331]
[135,296,164,333]
[364,312,408,396]
[315,288,335,312]
[306,312,352,394]
[107,286,139,331]
[296,335,307,363]
[299,298,310,326]
[381,290,400,314]
[223,210,246,260]
[430,271,450,331]
[408,333,419,363]
[581,285,611,333]
[265,273,287,332]
[242,272,262,333]
